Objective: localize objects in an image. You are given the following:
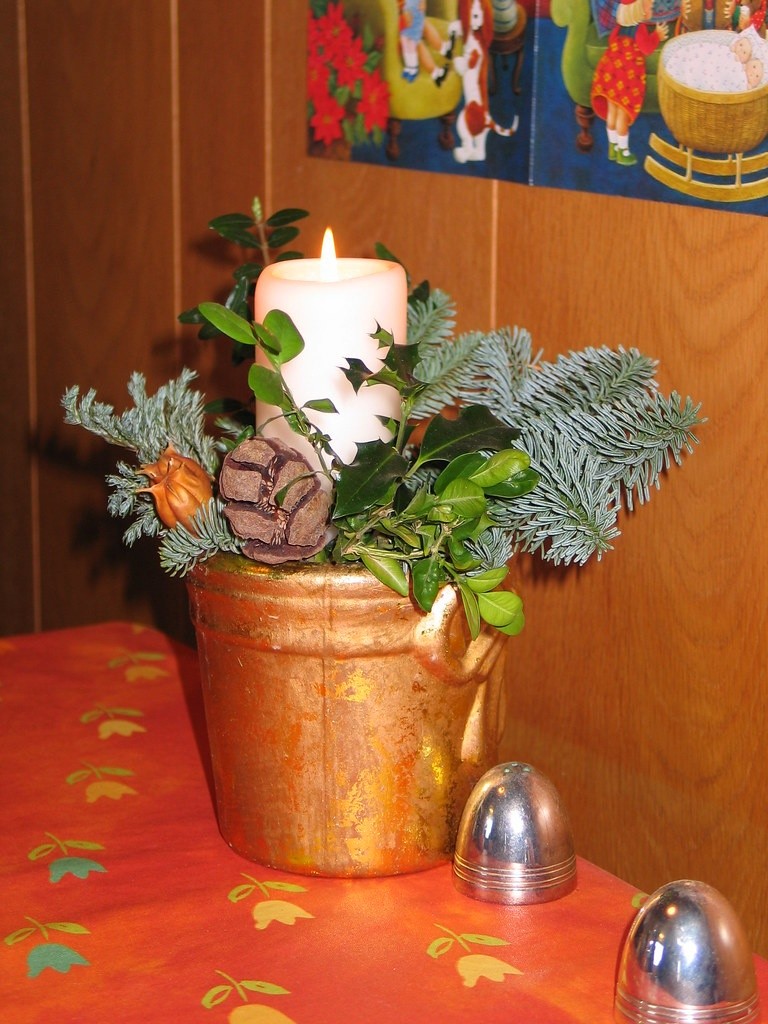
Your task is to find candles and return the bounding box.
[256,228,407,496]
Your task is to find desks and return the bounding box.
[0,620,768,1024]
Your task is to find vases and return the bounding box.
[168,543,510,878]
